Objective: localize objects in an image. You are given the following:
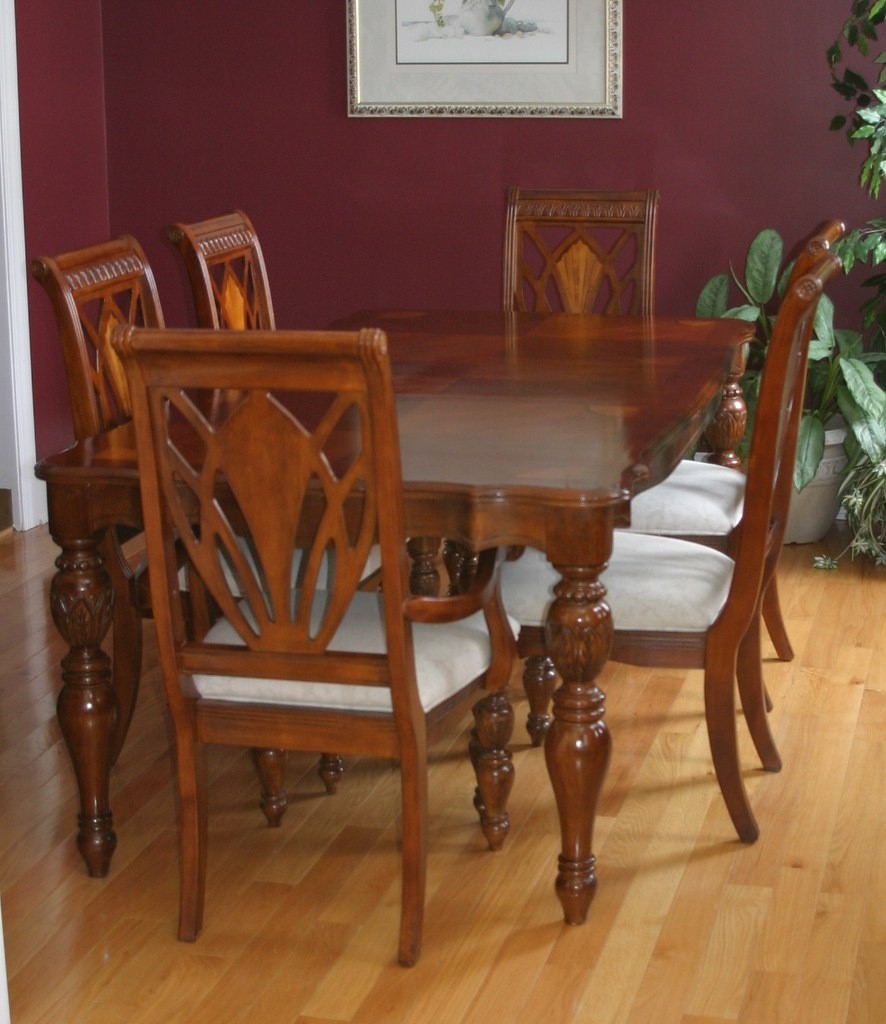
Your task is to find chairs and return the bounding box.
[31,234,411,797]
[160,209,278,330]
[106,317,522,965]
[492,254,842,842]
[499,185,661,316]
[633,218,845,711]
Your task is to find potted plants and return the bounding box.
[695,0,886,548]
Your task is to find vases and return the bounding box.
[347,0,624,119]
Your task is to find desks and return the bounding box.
[34,314,754,924]
